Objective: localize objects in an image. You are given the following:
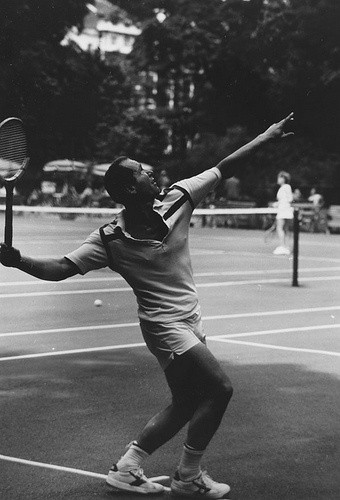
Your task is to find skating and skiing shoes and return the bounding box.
[274,248,290,255]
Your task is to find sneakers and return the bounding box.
[173,471,230,499]
[104,464,164,495]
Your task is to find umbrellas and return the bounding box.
[42,159,88,182]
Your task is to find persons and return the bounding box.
[0,111,295,500]
[273,170,294,255]
[308,187,325,214]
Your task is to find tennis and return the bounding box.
[94,299,103,307]
